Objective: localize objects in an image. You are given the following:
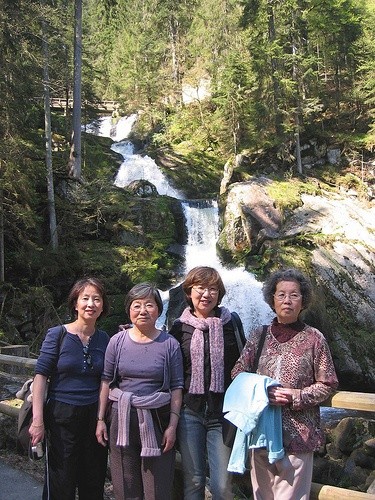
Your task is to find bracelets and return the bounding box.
[31,422,44,427]
[97,417,104,421]
[170,409,181,419]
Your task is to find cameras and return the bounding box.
[31,441,43,457]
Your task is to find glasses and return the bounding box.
[191,287,220,294]
[130,303,158,312]
[273,293,303,300]
[83,347,94,369]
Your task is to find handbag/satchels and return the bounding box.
[15,379,49,460]
[217,411,237,448]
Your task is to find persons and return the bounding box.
[230,269,340,500]
[118,266,248,500]
[28,277,111,500]
[95,282,185,500]
